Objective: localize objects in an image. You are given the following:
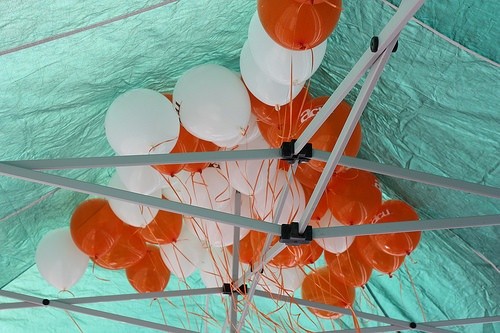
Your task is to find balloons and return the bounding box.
[239,0,343,106]
[35,227,90,292]
[70,62,422,319]
